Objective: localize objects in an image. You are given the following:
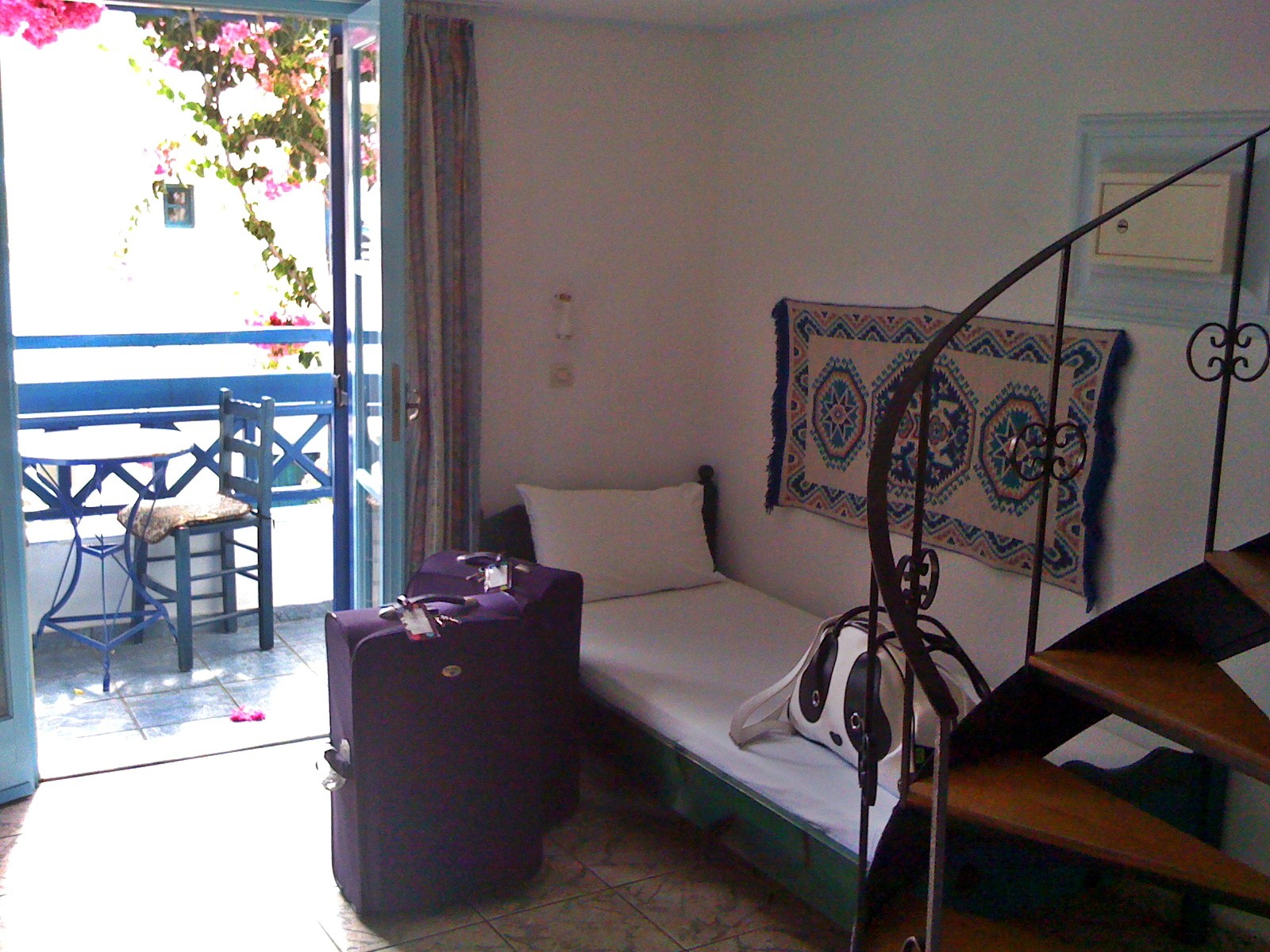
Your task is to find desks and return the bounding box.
[15,426,198,694]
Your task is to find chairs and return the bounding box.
[118,387,276,671]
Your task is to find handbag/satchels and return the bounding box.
[730,612,976,799]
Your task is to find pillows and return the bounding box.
[513,481,725,603]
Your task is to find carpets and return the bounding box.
[760,297,1132,615]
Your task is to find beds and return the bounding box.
[477,462,1005,952]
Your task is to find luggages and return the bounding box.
[405,547,584,824]
[323,590,544,920]
